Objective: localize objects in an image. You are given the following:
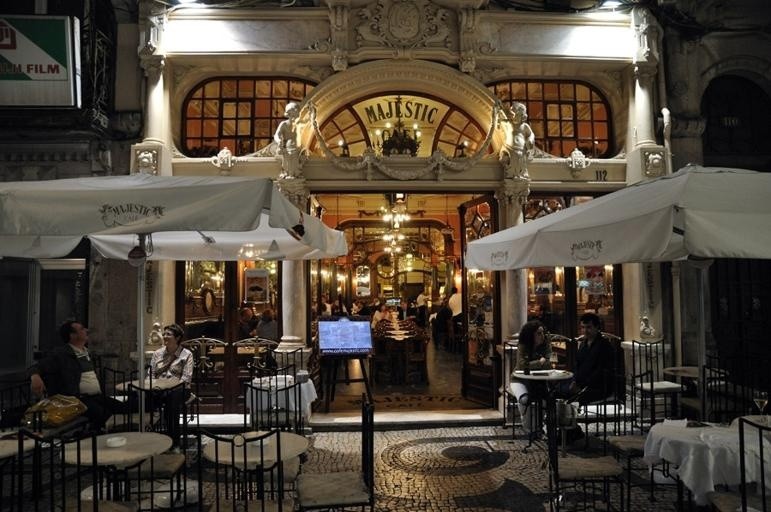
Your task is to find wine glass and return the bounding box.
[754,390,769,423]
[549,351,560,375]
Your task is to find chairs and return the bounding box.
[503,341,539,440]
[630,339,687,418]
[265,346,304,380]
[250,364,298,416]
[242,380,303,436]
[30,349,50,430]
[196,427,295,511]
[183,347,200,426]
[698,340,769,420]
[14,425,139,511]
[128,383,189,444]
[580,347,626,428]
[542,393,627,511]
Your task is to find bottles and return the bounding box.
[525,354,531,376]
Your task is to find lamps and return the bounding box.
[455,141,468,157]
[338,140,351,157]
[368,94,423,158]
[378,199,411,257]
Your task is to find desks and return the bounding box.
[1,429,37,466]
[114,378,185,408]
[732,413,771,451]
[203,430,309,496]
[370,317,430,385]
[245,374,317,414]
[606,423,682,510]
[646,419,757,496]
[63,431,172,502]
[662,364,730,417]
[512,368,574,432]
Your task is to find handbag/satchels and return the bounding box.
[24,394,87,428]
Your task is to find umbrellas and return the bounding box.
[1,173,349,428]
[465,163,771,422]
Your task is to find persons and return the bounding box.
[277,138,305,182]
[27,321,124,433]
[493,101,537,180]
[240,308,255,337]
[256,311,278,340]
[554,313,616,409]
[272,101,304,153]
[310,284,463,354]
[145,325,194,429]
[527,303,546,321]
[509,320,556,440]
